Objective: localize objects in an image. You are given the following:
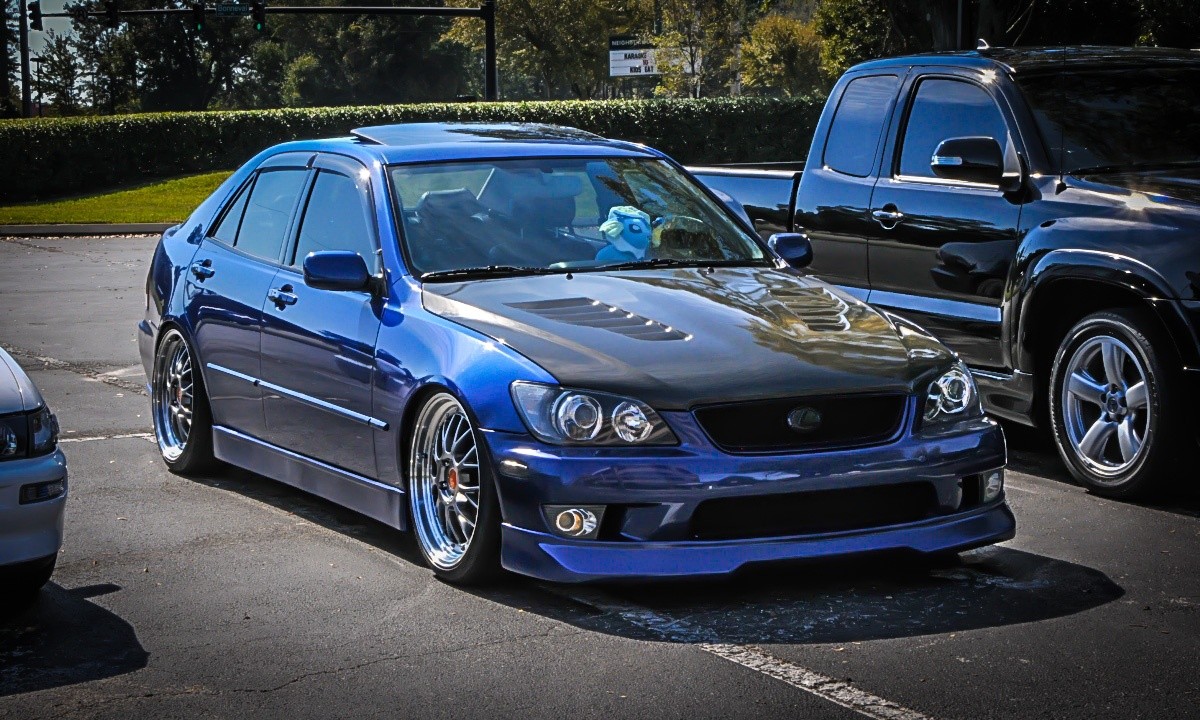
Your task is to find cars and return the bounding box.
[140,121,1017,586]
[1,348,69,597]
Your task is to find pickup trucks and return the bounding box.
[681,40,1200,499]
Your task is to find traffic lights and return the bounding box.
[105,1,119,31]
[253,1,265,32]
[27,0,43,30]
[192,3,205,34]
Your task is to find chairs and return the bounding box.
[416,188,486,268]
[492,189,595,263]
